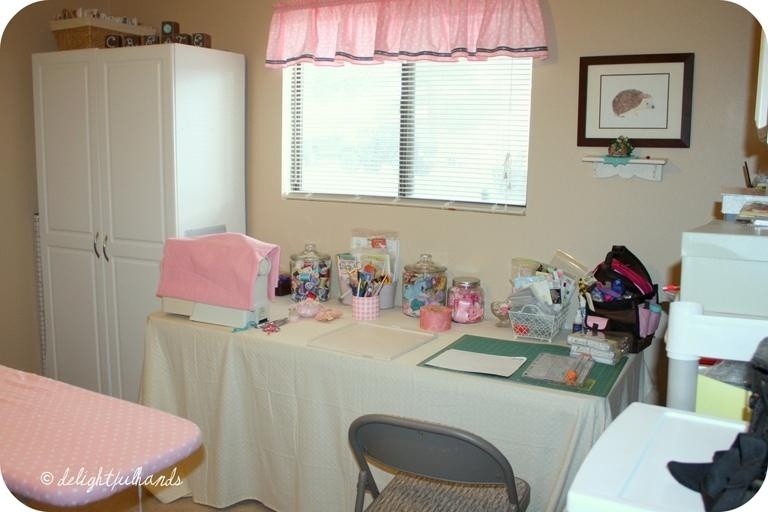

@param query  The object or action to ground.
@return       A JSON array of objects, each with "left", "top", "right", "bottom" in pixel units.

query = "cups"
[{"left": 338, "top": 277, "right": 396, "bottom": 317}]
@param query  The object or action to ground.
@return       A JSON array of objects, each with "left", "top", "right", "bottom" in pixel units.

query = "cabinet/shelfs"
[{"left": 29, "top": 44, "right": 247, "bottom": 403}]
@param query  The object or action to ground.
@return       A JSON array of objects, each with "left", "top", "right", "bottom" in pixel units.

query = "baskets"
[{"left": 507, "top": 303, "right": 572, "bottom": 343}]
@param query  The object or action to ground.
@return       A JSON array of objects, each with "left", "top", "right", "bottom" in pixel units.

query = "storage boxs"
[{"left": 47, "top": 18, "right": 157, "bottom": 50}]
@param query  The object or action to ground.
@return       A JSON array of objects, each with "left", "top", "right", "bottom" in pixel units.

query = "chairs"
[{"left": 346, "top": 413, "right": 532, "bottom": 511}]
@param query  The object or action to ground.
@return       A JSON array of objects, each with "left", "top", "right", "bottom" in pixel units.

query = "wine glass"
[{"left": 491, "top": 301, "right": 511, "bottom": 328}]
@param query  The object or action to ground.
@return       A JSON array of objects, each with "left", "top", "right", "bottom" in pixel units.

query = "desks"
[{"left": 142, "top": 292, "right": 641, "bottom": 510}]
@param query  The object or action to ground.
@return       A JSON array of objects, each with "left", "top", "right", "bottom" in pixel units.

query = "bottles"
[
  {"left": 402, "top": 254, "right": 484, "bottom": 324},
  {"left": 571, "top": 309, "right": 583, "bottom": 334},
  {"left": 289, "top": 243, "right": 333, "bottom": 304}
]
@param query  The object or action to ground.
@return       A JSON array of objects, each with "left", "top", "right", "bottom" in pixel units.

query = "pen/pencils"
[{"left": 351, "top": 271, "right": 387, "bottom": 295}]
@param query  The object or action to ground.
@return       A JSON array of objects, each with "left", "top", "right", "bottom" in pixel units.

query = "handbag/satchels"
[{"left": 582, "top": 246, "right": 661, "bottom": 354}]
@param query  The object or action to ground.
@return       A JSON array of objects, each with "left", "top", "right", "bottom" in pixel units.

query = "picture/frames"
[{"left": 576, "top": 51, "right": 698, "bottom": 150}]
